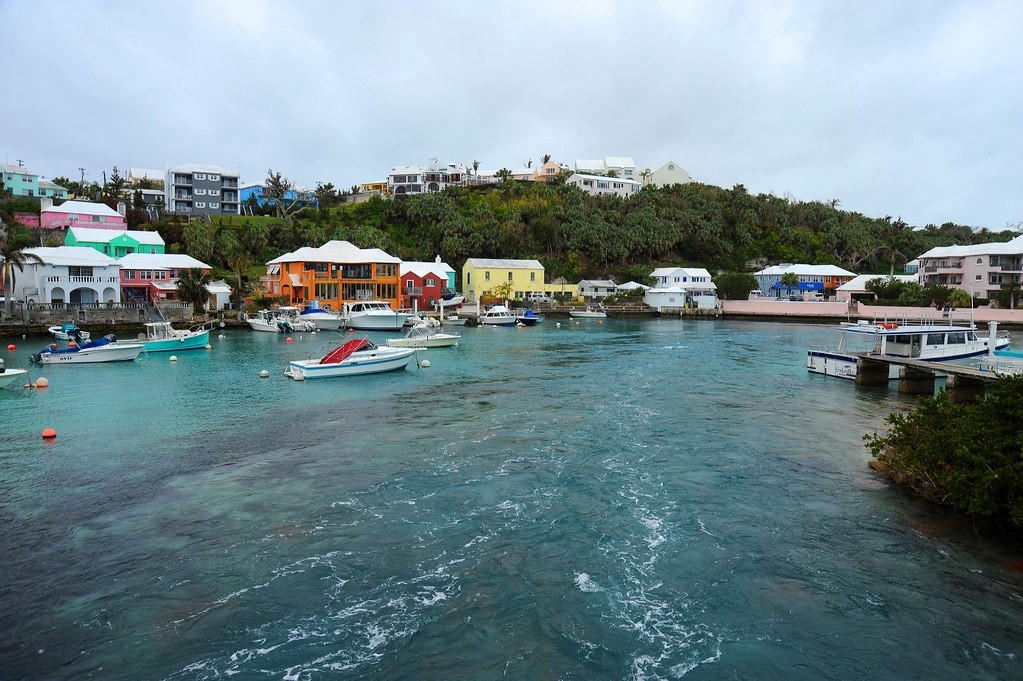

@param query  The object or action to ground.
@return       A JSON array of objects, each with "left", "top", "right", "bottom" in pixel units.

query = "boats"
[
  {"left": 48, "top": 325, "right": 91, "bottom": 340},
  {"left": 568, "top": 302, "right": 608, "bottom": 318},
  {"left": 244, "top": 296, "right": 416, "bottom": 334},
  {"left": 514, "top": 306, "right": 539, "bottom": 326},
  {"left": 430, "top": 288, "right": 465, "bottom": 307},
  {"left": 283, "top": 329, "right": 417, "bottom": 381},
  {"left": 474, "top": 303, "right": 518, "bottom": 327},
  {"left": 0, "top": 358, "right": 28, "bottom": 391},
  {"left": 385, "top": 319, "right": 462, "bottom": 348},
  {"left": 807, "top": 264, "right": 1011, "bottom": 381},
  {"left": 107, "top": 295, "right": 210, "bottom": 352},
  {"left": 28, "top": 319, "right": 146, "bottom": 365}
]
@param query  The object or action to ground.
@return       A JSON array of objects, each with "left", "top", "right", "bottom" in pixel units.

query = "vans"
[{"left": 526, "top": 292, "right": 551, "bottom": 304}]
